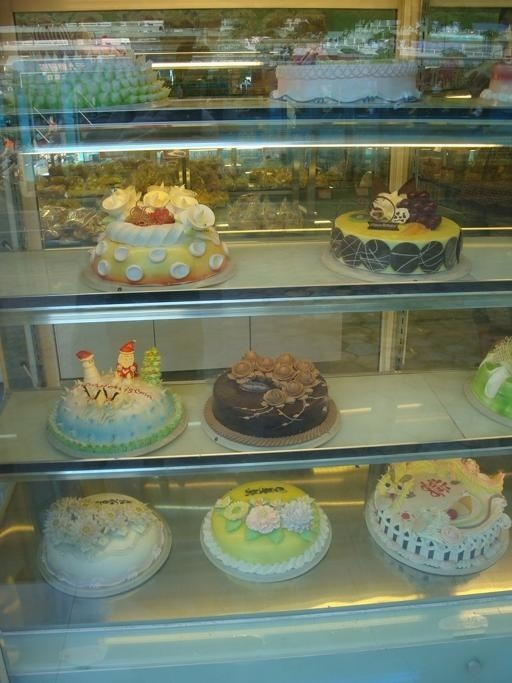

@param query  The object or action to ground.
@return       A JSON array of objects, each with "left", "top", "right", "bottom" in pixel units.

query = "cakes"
[
  {"left": 34, "top": 493, "right": 172, "bottom": 598},
  {"left": 1, "top": 47, "right": 172, "bottom": 116},
  {"left": 272, "top": 60, "right": 422, "bottom": 106},
  {"left": 463, "top": 335, "right": 511, "bottom": 428},
  {"left": 45, "top": 338, "right": 189, "bottom": 458},
  {"left": 326, "top": 189, "right": 463, "bottom": 279},
  {"left": 364, "top": 458, "right": 511, "bottom": 577},
  {"left": 203, "top": 351, "right": 338, "bottom": 448},
  {"left": 200, "top": 480, "right": 332, "bottom": 584},
  {"left": 478, "top": 64, "right": 512, "bottom": 103},
  {"left": 78, "top": 180, "right": 238, "bottom": 293}
]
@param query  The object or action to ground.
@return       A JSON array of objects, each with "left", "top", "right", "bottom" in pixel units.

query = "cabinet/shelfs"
[{"left": 0, "top": 108, "right": 512, "bottom": 681}]
